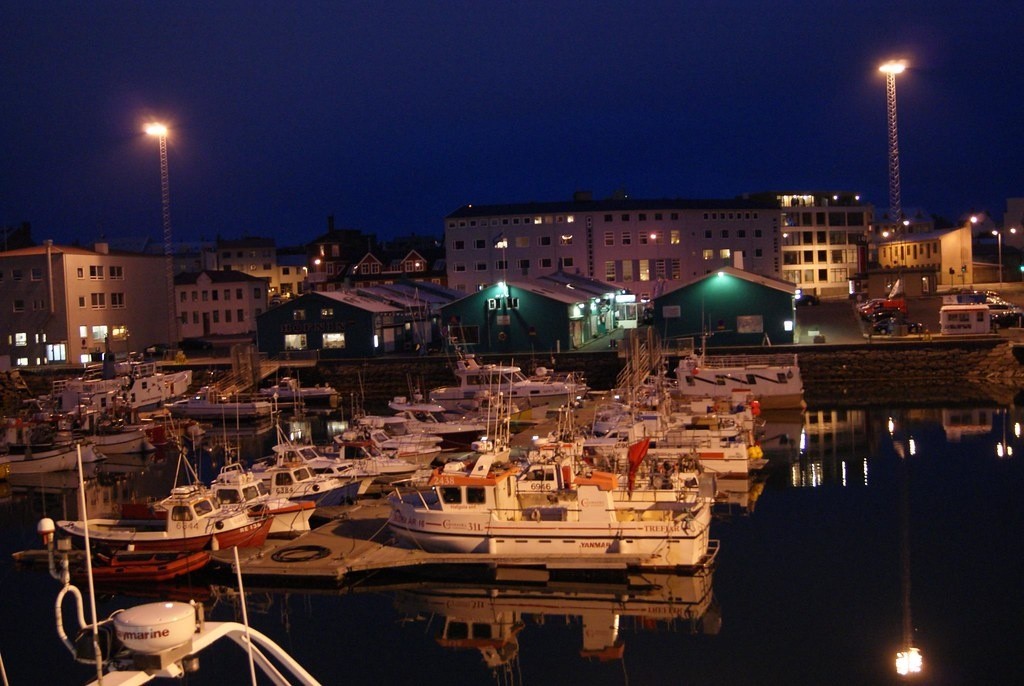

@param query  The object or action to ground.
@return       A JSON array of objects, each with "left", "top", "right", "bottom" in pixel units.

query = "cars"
[
  {"left": 794, "top": 295, "right": 819, "bottom": 307},
  {"left": 857, "top": 296, "right": 929, "bottom": 334},
  {"left": 950, "top": 289, "right": 1024, "bottom": 328}
]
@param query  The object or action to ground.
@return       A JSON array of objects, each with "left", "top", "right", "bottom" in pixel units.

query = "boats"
[{"left": 0, "top": 351, "right": 802, "bottom": 686}]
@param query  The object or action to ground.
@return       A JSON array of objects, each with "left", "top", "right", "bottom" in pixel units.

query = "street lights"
[
  {"left": 496, "top": 241, "right": 507, "bottom": 281},
  {"left": 122, "top": 330, "right": 131, "bottom": 364},
  {"left": 993, "top": 230, "right": 1002, "bottom": 282},
  {"left": 302, "top": 267, "right": 308, "bottom": 289}
]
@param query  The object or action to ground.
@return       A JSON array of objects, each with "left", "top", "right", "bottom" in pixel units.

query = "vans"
[{"left": 860, "top": 296, "right": 906, "bottom": 316}]
